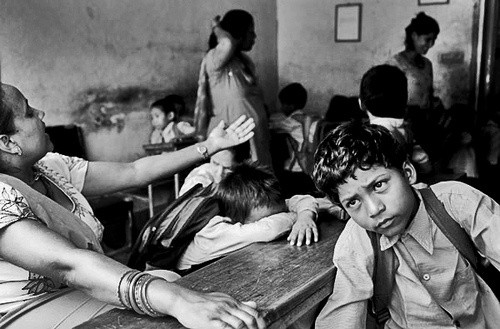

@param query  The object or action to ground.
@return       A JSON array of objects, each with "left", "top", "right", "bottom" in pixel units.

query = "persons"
[
  {"left": 197, "top": 9, "right": 272, "bottom": 174},
  {"left": 384, "top": 11, "right": 500, "bottom": 190},
  {"left": 0, "top": 83, "right": 266, "bottom": 329},
  {"left": 310, "top": 121, "right": 500, "bottom": 329},
  {"left": 268, "top": 82, "right": 311, "bottom": 173},
  {"left": 315, "top": 65, "right": 431, "bottom": 221},
  {"left": 151, "top": 93, "right": 195, "bottom": 144},
  {"left": 177, "top": 150, "right": 239, "bottom": 197},
  {"left": 127, "top": 162, "right": 320, "bottom": 277}
]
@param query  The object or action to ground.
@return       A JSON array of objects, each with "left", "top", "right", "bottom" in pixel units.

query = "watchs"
[{"left": 193, "top": 143, "right": 212, "bottom": 158}]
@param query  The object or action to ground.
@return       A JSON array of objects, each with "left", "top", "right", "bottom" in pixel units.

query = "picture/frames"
[{"left": 335, "top": 2, "right": 362, "bottom": 42}]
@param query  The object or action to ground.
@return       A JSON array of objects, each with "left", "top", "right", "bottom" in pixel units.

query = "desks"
[{"left": 69, "top": 214, "right": 360, "bottom": 329}]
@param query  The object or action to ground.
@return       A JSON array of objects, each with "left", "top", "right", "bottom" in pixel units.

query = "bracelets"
[
  {"left": 212, "top": 22, "right": 220, "bottom": 31},
  {"left": 116, "top": 268, "right": 167, "bottom": 317}
]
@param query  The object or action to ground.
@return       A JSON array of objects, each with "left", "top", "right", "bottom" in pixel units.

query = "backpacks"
[
  {"left": 287, "top": 111, "right": 336, "bottom": 176},
  {"left": 126, "top": 182, "right": 221, "bottom": 272}
]
